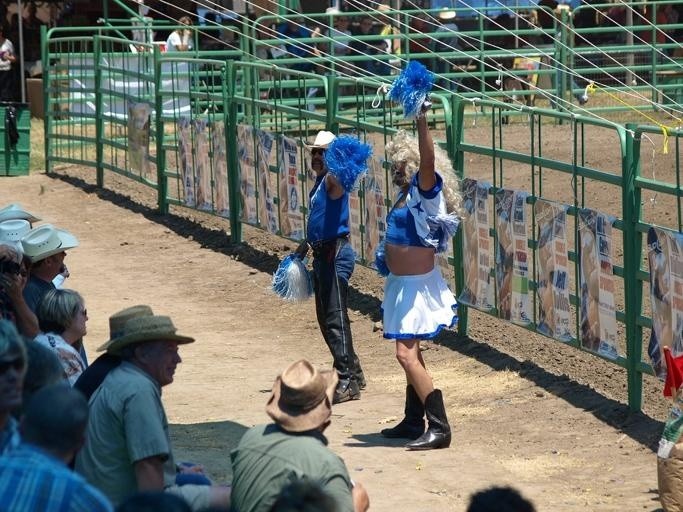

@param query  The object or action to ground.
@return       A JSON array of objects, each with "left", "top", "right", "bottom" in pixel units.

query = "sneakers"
[{"left": 334, "top": 388, "right": 360, "bottom": 404}]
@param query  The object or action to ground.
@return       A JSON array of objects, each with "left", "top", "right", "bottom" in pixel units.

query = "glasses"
[{"left": 311, "top": 148, "right": 324, "bottom": 155}]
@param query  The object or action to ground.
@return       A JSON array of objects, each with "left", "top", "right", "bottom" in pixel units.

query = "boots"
[
  {"left": 381, "top": 384, "right": 425, "bottom": 437},
  {"left": 406, "top": 389, "right": 451, "bottom": 450}
]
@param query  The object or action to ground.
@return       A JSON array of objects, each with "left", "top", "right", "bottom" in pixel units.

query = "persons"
[
  {"left": 229, "top": 359, "right": 369, "bottom": 512},
  {"left": 74, "top": 305, "right": 231, "bottom": 511},
  {"left": 273, "top": 4, "right": 401, "bottom": 111},
  {"left": 165, "top": 16, "right": 193, "bottom": 52},
  {"left": 201, "top": 12, "right": 220, "bottom": 40},
  {"left": 1, "top": 26, "right": 15, "bottom": 99},
  {"left": 273, "top": 129, "right": 374, "bottom": 405},
  {"left": 410, "top": 5, "right": 459, "bottom": 92},
  {"left": 1, "top": 203, "right": 113, "bottom": 510},
  {"left": 371, "top": 63, "right": 466, "bottom": 452},
  {"left": 569, "top": 0, "right": 679, "bottom": 84}
]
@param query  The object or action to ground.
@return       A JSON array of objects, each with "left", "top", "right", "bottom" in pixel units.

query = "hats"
[
  {"left": 301, "top": 130, "right": 337, "bottom": 149},
  {"left": 439, "top": 7, "right": 456, "bottom": 19},
  {"left": 375, "top": 5, "right": 390, "bottom": 11},
  {"left": 325, "top": 7, "right": 340, "bottom": 13},
  {"left": 555, "top": 4, "right": 576, "bottom": 16},
  {"left": 265, "top": 356, "right": 339, "bottom": 433},
  {"left": 0, "top": 203, "right": 196, "bottom": 351}
]
